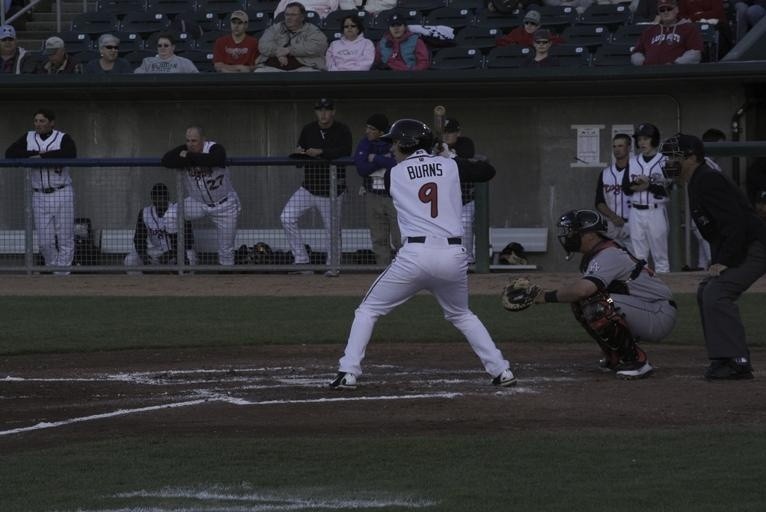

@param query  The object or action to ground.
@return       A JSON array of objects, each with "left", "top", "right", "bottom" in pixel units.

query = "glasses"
[
  {"left": 103, "top": 46, "right": 120, "bottom": 49},
  {"left": 535, "top": 40, "right": 550, "bottom": 44}
]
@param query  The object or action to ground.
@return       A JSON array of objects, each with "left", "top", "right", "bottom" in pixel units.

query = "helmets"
[
  {"left": 380, "top": 119, "right": 433, "bottom": 154},
  {"left": 661, "top": 134, "right": 705, "bottom": 178},
  {"left": 557, "top": 209, "right": 608, "bottom": 261},
  {"left": 632, "top": 123, "right": 661, "bottom": 149}
]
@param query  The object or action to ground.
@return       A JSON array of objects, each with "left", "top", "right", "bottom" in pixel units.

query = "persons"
[
  {"left": 503, "top": 210, "right": 677, "bottom": 380},
  {"left": 658, "top": 133, "right": 766, "bottom": 380},
  {"left": 331, "top": 119, "right": 516, "bottom": 389}
]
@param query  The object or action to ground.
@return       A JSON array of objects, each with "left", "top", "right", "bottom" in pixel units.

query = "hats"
[
  {"left": 389, "top": 13, "right": 405, "bottom": 25},
  {"left": 523, "top": 10, "right": 542, "bottom": 24},
  {"left": 444, "top": 119, "right": 460, "bottom": 133},
  {"left": 42, "top": 36, "right": 64, "bottom": 56},
  {"left": 313, "top": 98, "right": 334, "bottom": 109},
  {"left": 532, "top": 28, "right": 551, "bottom": 40},
  {"left": 0, "top": 25, "right": 17, "bottom": 41},
  {"left": 229, "top": 10, "right": 249, "bottom": 23},
  {"left": 657, "top": 1, "right": 678, "bottom": 8}
]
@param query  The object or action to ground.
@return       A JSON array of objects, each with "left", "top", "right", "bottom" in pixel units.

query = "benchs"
[{"left": 2, "top": 227, "right": 550, "bottom": 256}]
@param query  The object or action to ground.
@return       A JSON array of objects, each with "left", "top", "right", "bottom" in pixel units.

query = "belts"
[
  {"left": 632, "top": 203, "right": 658, "bottom": 209},
  {"left": 407, "top": 236, "right": 461, "bottom": 245},
  {"left": 208, "top": 197, "right": 228, "bottom": 207},
  {"left": 33, "top": 186, "right": 65, "bottom": 193}
]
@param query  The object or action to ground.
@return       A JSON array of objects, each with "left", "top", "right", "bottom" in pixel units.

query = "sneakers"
[
  {"left": 598, "top": 356, "right": 615, "bottom": 372},
  {"left": 615, "top": 361, "right": 651, "bottom": 379},
  {"left": 705, "top": 359, "right": 753, "bottom": 381},
  {"left": 490, "top": 369, "right": 517, "bottom": 387},
  {"left": 330, "top": 372, "right": 359, "bottom": 390}
]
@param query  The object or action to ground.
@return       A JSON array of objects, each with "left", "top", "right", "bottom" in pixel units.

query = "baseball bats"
[{"left": 434, "top": 106, "right": 445, "bottom": 137}]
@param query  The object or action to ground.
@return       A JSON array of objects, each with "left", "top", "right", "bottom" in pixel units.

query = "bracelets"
[{"left": 544, "top": 289, "right": 559, "bottom": 303}]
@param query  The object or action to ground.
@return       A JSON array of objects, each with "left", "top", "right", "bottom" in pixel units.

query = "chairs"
[{"left": 2, "top": 1, "right": 764, "bottom": 73}]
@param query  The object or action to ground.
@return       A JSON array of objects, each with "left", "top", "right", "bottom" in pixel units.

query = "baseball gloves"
[{"left": 500, "top": 277, "right": 544, "bottom": 311}]
